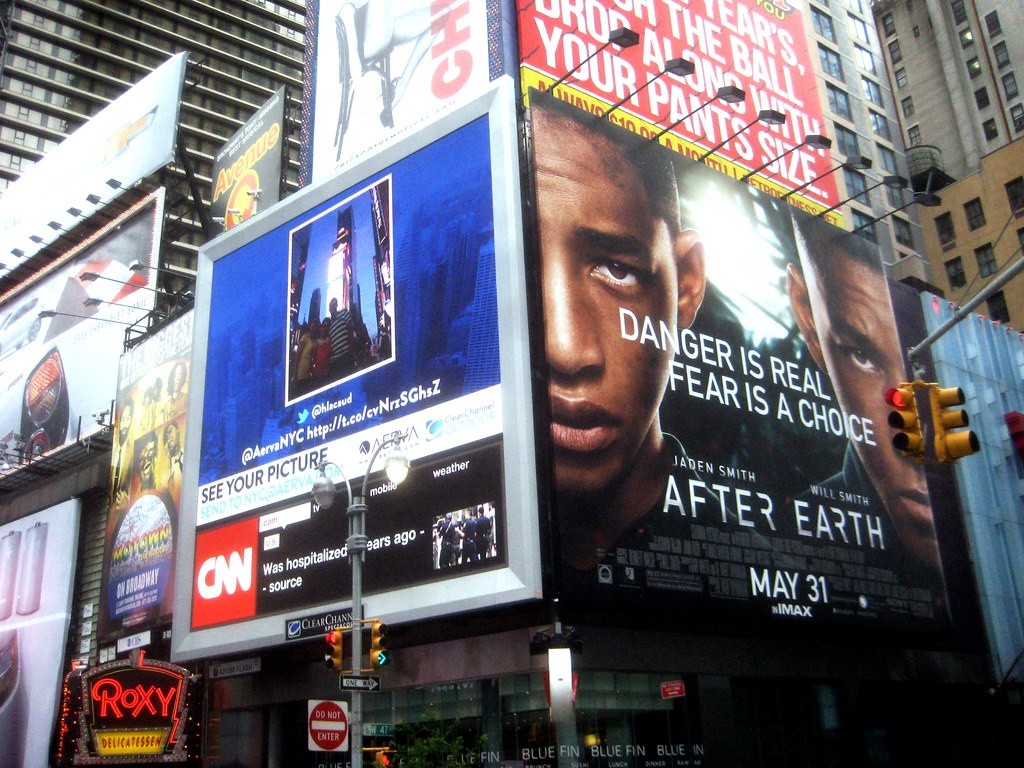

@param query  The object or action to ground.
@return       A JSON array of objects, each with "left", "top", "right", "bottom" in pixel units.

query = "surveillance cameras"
[{"left": 92, "top": 409, "right": 109, "bottom": 417}]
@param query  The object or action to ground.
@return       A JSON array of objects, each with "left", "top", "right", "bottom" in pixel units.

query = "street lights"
[{"left": 311, "top": 433, "right": 412, "bottom": 768}]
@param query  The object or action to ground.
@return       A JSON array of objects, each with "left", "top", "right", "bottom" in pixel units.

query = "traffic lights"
[
  {"left": 370, "top": 623, "right": 391, "bottom": 669},
  {"left": 884, "top": 382, "right": 923, "bottom": 457},
  {"left": 928, "top": 385, "right": 980, "bottom": 465},
  {"left": 325, "top": 630, "right": 343, "bottom": 672}
]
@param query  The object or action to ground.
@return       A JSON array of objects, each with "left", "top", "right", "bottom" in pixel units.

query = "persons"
[
  {"left": 292, "top": 315, "right": 318, "bottom": 399},
  {"left": 313, "top": 318, "right": 331, "bottom": 388},
  {"left": 326, "top": 298, "right": 353, "bottom": 384},
  {"left": 110, "top": 361, "right": 189, "bottom": 510},
  {"left": 786, "top": 209, "right": 942, "bottom": 567},
  {"left": 529, "top": 89, "right": 820, "bottom": 628},
  {"left": 438, "top": 507, "right": 491, "bottom": 568}
]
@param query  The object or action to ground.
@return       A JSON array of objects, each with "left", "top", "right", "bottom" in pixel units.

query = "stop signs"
[{"left": 307, "top": 699, "right": 349, "bottom": 752}]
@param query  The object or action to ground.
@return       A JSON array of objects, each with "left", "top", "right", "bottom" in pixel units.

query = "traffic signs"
[{"left": 339, "top": 674, "right": 382, "bottom": 694}]
